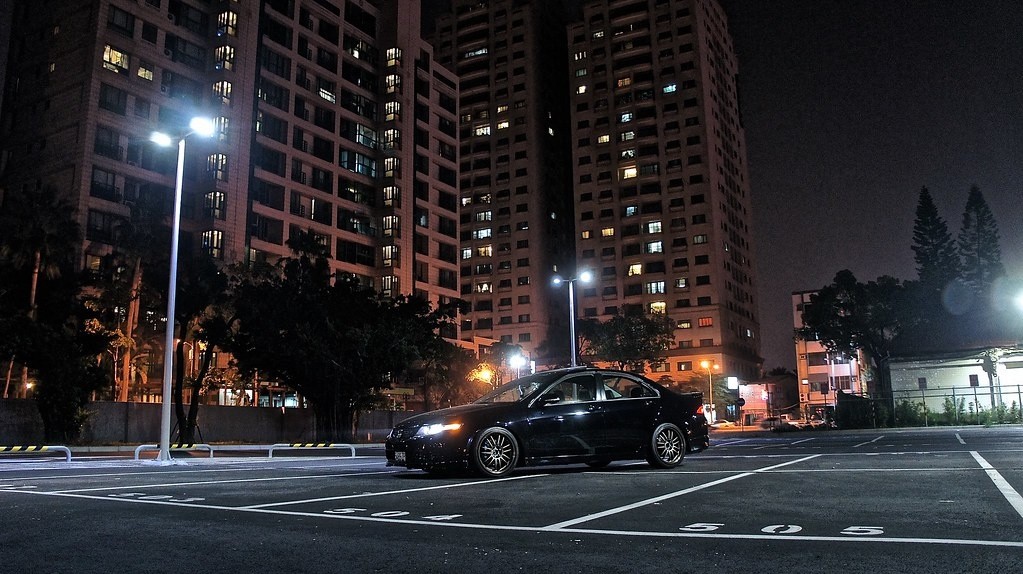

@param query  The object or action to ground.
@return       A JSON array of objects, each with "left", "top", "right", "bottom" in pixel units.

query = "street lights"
[
  {"left": 554, "top": 271, "right": 592, "bottom": 401},
  {"left": 512, "top": 356, "right": 524, "bottom": 390},
  {"left": 824, "top": 357, "right": 844, "bottom": 412},
  {"left": 702, "top": 361, "right": 719, "bottom": 422},
  {"left": 149, "top": 115, "right": 211, "bottom": 461}
]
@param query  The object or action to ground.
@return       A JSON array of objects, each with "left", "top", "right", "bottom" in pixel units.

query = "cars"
[
  {"left": 708, "top": 419, "right": 735, "bottom": 430},
  {"left": 761, "top": 416, "right": 789, "bottom": 430},
  {"left": 385, "top": 363, "right": 710, "bottom": 479},
  {"left": 784, "top": 416, "right": 838, "bottom": 429}
]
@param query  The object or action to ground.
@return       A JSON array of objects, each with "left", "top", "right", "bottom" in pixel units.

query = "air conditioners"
[{"left": 800, "top": 393, "right": 808, "bottom": 402}]
